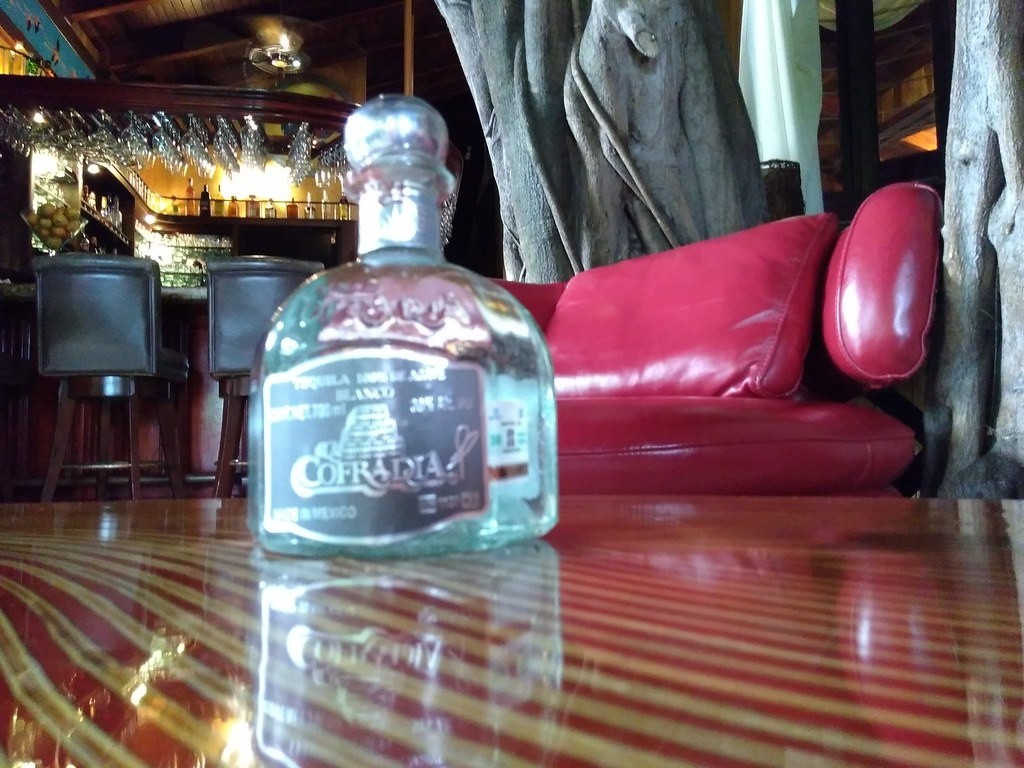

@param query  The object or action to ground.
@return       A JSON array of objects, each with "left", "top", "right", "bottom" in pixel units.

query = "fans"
[{"left": 249, "top": 35, "right": 308, "bottom": 75}]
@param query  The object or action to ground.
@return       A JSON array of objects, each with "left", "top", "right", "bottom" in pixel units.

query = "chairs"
[
  {"left": 207, "top": 256, "right": 325, "bottom": 500},
  {"left": 33, "top": 252, "right": 190, "bottom": 500}
]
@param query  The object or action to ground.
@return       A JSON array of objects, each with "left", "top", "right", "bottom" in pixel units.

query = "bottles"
[
  {"left": 228, "top": 196, "right": 240, "bottom": 217},
  {"left": 246, "top": 195, "right": 260, "bottom": 219},
  {"left": 287, "top": 198, "right": 298, "bottom": 219},
  {"left": 170, "top": 195, "right": 181, "bottom": 215},
  {"left": 214, "top": 185, "right": 225, "bottom": 216},
  {"left": 198, "top": 183, "right": 211, "bottom": 216},
  {"left": 334, "top": 192, "right": 350, "bottom": 220},
  {"left": 79, "top": 184, "right": 124, "bottom": 255},
  {"left": 183, "top": 176, "right": 196, "bottom": 215},
  {"left": 303, "top": 192, "right": 317, "bottom": 220},
  {"left": 234, "top": 94, "right": 561, "bottom": 558},
  {"left": 265, "top": 198, "right": 276, "bottom": 218},
  {"left": 320, "top": 189, "right": 332, "bottom": 220}
]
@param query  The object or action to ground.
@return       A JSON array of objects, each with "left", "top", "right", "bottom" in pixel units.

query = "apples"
[{"left": 26, "top": 203, "right": 81, "bottom": 249}]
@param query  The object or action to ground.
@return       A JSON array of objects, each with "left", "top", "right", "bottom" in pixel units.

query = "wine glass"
[{"left": 1, "top": 102, "right": 348, "bottom": 188}]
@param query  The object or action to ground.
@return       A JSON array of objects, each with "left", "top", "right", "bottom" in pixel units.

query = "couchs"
[{"left": 489, "top": 181, "right": 943, "bottom": 496}]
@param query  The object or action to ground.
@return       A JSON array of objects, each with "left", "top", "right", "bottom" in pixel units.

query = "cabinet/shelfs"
[{"left": 0, "top": 146, "right": 137, "bottom": 284}]
